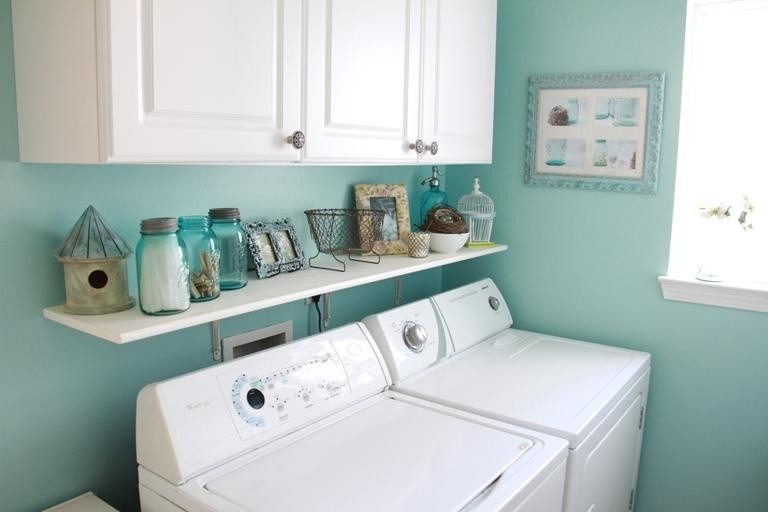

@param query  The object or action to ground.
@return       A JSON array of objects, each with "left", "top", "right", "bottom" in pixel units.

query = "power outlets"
[{"left": 304, "top": 295, "right": 323, "bottom": 304}]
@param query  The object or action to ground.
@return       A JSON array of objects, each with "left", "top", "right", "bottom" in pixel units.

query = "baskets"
[{"left": 305, "top": 208, "right": 386, "bottom": 254}]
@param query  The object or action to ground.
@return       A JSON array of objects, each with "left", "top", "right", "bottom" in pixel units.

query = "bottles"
[
  {"left": 134, "top": 217, "right": 190, "bottom": 316},
  {"left": 177, "top": 214, "right": 221, "bottom": 303},
  {"left": 208, "top": 207, "right": 248, "bottom": 292},
  {"left": 593, "top": 139, "right": 607, "bottom": 168},
  {"left": 566, "top": 98, "right": 580, "bottom": 125}
]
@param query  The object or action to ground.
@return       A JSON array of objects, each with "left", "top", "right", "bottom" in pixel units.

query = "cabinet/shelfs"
[{"left": 11, "top": 0, "right": 499, "bottom": 169}]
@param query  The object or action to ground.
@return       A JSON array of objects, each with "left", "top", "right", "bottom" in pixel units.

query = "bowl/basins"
[{"left": 425, "top": 229, "right": 470, "bottom": 254}]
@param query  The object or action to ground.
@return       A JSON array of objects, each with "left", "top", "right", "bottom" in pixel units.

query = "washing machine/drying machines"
[
  {"left": 135, "top": 320, "right": 569, "bottom": 512},
  {"left": 361, "top": 276, "right": 653, "bottom": 512}
]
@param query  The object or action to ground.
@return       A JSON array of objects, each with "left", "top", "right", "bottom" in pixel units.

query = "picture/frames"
[
  {"left": 353, "top": 182, "right": 412, "bottom": 257},
  {"left": 242, "top": 217, "right": 308, "bottom": 280},
  {"left": 521, "top": 69, "right": 667, "bottom": 195}
]
